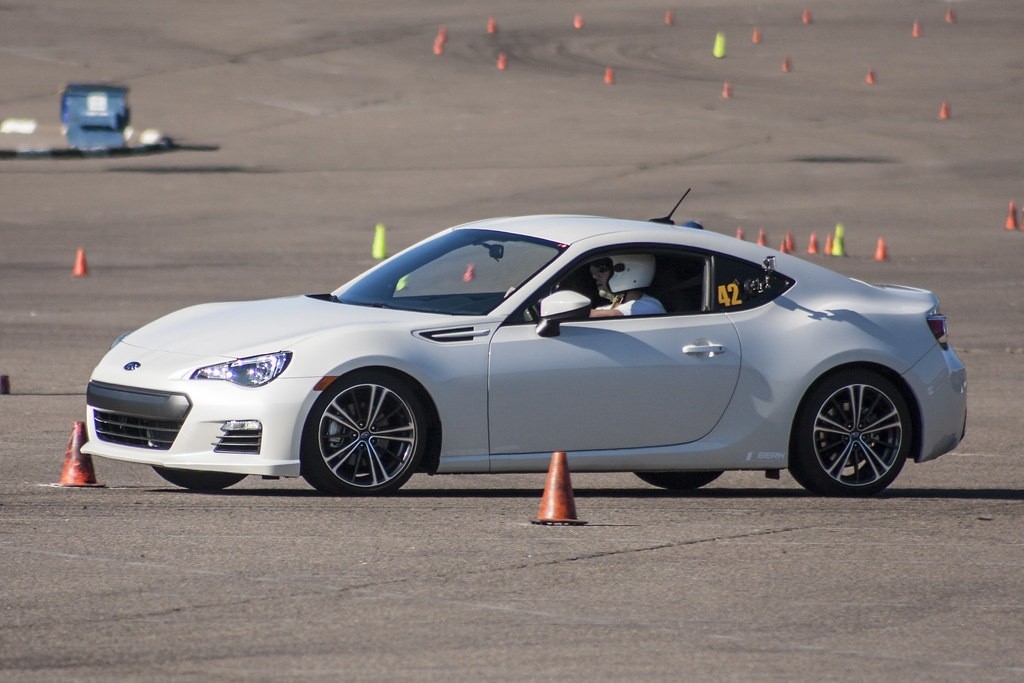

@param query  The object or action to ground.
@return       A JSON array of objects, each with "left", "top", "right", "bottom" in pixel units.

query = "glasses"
[{"left": 598, "top": 263, "right": 611, "bottom": 272}]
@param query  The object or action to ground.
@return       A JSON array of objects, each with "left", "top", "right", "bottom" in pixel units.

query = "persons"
[{"left": 588, "top": 252, "right": 666, "bottom": 317}]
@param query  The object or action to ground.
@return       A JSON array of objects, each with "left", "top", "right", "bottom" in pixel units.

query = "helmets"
[{"left": 591, "top": 256, "right": 655, "bottom": 294}]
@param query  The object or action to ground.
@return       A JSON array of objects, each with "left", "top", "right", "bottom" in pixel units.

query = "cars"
[{"left": 78, "top": 212, "right": 969, "bottom": 498}]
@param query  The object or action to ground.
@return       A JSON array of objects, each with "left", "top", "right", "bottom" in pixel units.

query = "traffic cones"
[
  {"left": 722, "top": 82, "right": 733, "bottom": 99},
  {"left": 56, "top": 420, "right": 106, "bottom": 488},
  {"left": 945, "top": 7, "right": 955, "bottom": 23},
  {"left": 371, "top": 223, "right": 386, "bottom": 259},
  {"left": 573, "top": 13, "right": 583, "bottom": 29},
  {"left": 939, "top": 98, "right": 950, "bottom": 119},
  {"left": 487, "top": 18, "right": 497, "bottom": 34},
  {"left": 432, "top": 25, "right": 449, "bottom": 54},
  {"left": 604, "top": 67, "right": 615, "bottom": 83},
  {"left": 72, "top": 247, "right": 88, "bottom": 276},
  {"left": 736, "top": 222, "right": 846, "bottom": 257},
  {"left": 712, "top": 8, "right": 812, "bottom": 72},
  {"left": 874, "top": 235, "right": 887, "bottom": 261},
  {"left": 664, "top": 9, "right": 674, "bottom": 25},
  {"left": 497, "top": 53, "right": 507, "bottom": 70},
  {"left": 912, "top": 17, "right": 922, "bottom": 37},
  {"left": 1004, "top": 200, "right": 1024, "bottom": 231},
  {"left": 865, "top": 71, "right": 876, "bottom": 85},
  {"left": 529, "top": 449, "right": 589, "bottom": 527}
]
